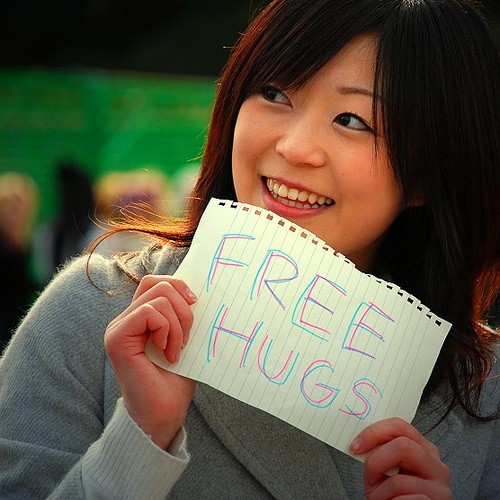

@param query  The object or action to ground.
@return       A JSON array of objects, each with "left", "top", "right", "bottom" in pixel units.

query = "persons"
[
  {"left": 1, "top": 0, "right": 500, "bottom": 500},
  {"left": 1, "top": 160, "right": 170, "bottom": 358}
]
